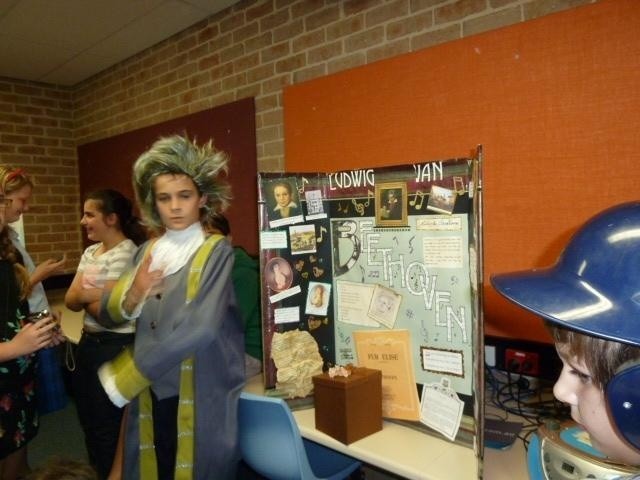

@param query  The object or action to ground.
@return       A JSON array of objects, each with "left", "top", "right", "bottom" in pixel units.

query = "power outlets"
[{"left": 485, "top": 345, "right": 540, "bottom": 392}]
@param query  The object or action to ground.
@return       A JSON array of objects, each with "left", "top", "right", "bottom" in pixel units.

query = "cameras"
[{"left": 26, "top": 309, "right": 61, "bottom": 332}]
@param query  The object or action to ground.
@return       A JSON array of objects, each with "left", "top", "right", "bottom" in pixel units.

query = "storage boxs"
[{"left": 312, "top": 364, "right": 385, "bottom": 447}]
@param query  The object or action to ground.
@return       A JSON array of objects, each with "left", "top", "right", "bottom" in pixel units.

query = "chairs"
[{"left": 234, "top": 391, "right": 364, "bottom": 480}]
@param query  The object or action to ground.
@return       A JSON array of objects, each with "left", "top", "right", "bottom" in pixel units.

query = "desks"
[{"left": 240, "top": 362, "right": 486, "bottom": 478}]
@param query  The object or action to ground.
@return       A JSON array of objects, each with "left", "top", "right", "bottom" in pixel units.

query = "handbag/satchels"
[{"left": 60, "top": 307, "right": 85, "bottom": 345}]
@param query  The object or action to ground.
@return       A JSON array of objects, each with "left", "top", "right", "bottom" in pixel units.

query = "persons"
[
  {"left": 267, "top": 179, "right": 300, "bottom": 220},
  {"left": 0, "top": 163, "right": 98, "bottom": 479},
  {"left": 63, "top": 187, "right": 140, "bottom": 479},
  {"left": 310, "top": 285, "right": 324, "bottom": 307},
  {"left": 268, "top": 260, "right": 291, "bottom": 291},
  {"left": 382, "top": 189, "right": 399, "bottom": 219},
  {"left": 201, "top": 210, "right": 262, "bottom": 362},
  {"left": 97, "top": 135, "right": 246, "bottom": 480}
]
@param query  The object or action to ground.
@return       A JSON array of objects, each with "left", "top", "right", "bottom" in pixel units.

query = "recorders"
[{"left": 527, "top": 419, "right": 640, "bottom": 480}]
